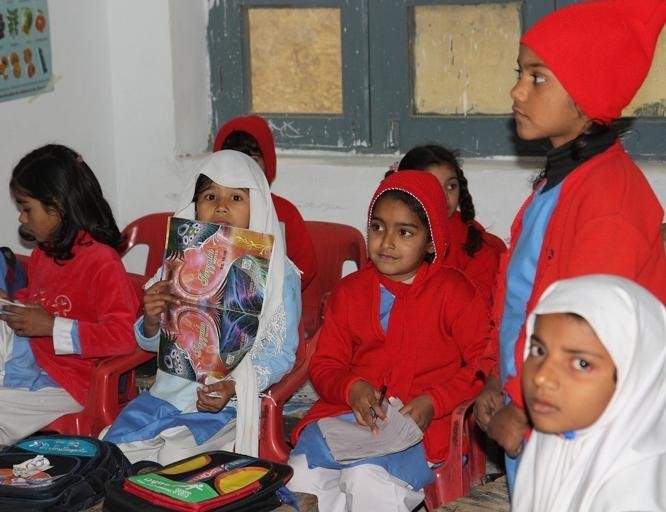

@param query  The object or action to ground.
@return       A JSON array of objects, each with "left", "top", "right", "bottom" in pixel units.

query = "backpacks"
[
  {"left": 101, "top": 449, "right": 301, "bottom": 512},
  {"left": 0, "top": 434, "right": 131, "bottom": 512}
]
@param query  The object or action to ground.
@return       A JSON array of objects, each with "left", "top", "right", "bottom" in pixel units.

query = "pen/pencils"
[{"left": 373, "top": 385, "right": 388, "bottom": 426}]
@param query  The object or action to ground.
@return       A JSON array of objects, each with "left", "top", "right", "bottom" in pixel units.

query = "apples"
[{"left": 36, "top": 8, "right": 45, "bottom": 32}]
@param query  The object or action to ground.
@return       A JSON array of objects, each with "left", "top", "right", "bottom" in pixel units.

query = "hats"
[{"left": 520, "top": 0, "right": 666, "bottom": 124}]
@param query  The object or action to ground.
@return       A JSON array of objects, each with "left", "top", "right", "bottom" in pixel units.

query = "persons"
[
  {"left": 210, "top": 114, "right": 317, "bottom": 291},
  {"left": 509, "top": 272, "right": 666, "bottom": 512},
  {"left": 379, "top": 144, "right": 507, "bottom": 313},
  {"left": 0, "top": 144, "right": 141, "bottom": 447},
  {"left": 95, "top": 148, "right": 304, "bottom": 467},
  {"left": 286, "top": 171, "right": 494, "bottom": 512},
  {"left": 472, "top": 0, "right": 666, "bottom": 507}
]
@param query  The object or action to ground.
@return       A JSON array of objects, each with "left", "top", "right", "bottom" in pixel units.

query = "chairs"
[
  {"left": 424, "top": 227, "right": 506, "bottom": 510},
  {"left": 260, "top": 220, "right": 368, "bottom": 464},
  {"left": 38, "top": 213, "right": 168, "bottom": 436}
]
